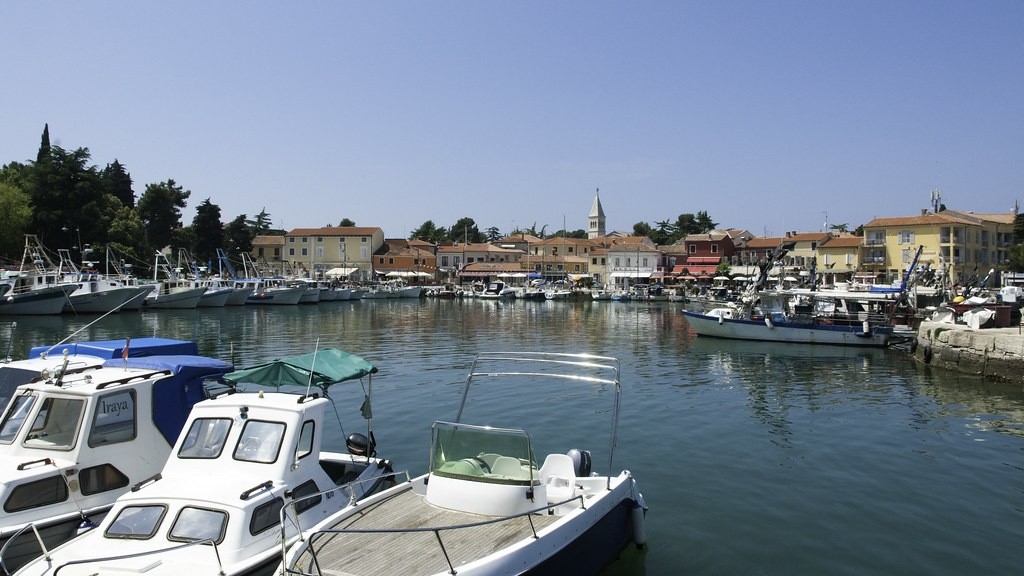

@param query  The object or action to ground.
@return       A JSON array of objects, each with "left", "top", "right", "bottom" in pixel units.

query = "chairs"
[
  {"left": 491, "top": 456, "right": 522, "bottom": 478},
  {"left": 536, "top": 453, "right": 577, "bottom": 504}
]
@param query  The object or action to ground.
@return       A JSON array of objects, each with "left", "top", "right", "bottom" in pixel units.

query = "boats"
[
  {"left": 0, "top": 226, "right": 1023, "bottom": 348},
  {"left": 267, "top": 351, "right": 648, "bottom": 576},
  {"left": 0, "top": 336, "right": 200, "bottom": 436},
  {"left": 0, "top": 356, "right": 236, "bottom": 561},
  {"left": 0, "top": 348, "right": 396, "bottom": 576}
]
